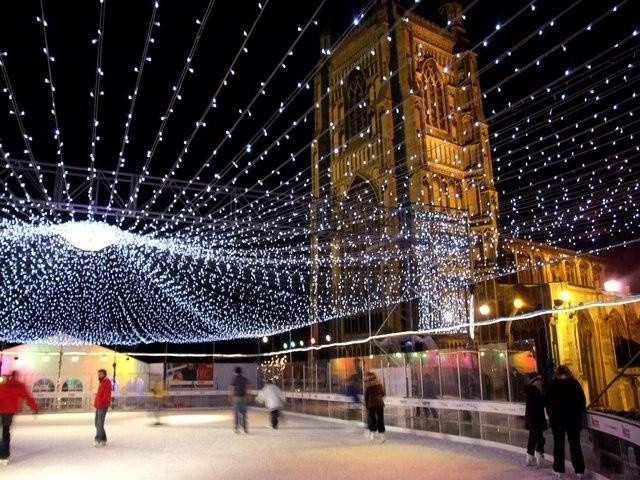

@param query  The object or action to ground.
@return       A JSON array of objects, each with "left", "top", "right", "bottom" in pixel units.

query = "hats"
[{"left": 527, "top": 371, "right": 541, "bottom": 385}]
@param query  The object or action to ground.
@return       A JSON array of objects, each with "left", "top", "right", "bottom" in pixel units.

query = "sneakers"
[{"left": 525, "top": 451, "right": 587, "bottom": 479}]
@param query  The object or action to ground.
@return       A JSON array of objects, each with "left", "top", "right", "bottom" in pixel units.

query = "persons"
[
  {"left": 227, "top": 366, "right": 250, "bottom": 433},
  {"left": 166, "top": 363, "right": 199, "bottom": 389},
  {"left": 0, "top": 369, "right": 40, "bottom": 460},
  {"left": 523, "top": 370, "right": 553, "bottom": 468},
  {"left": 148, "top": 374, "right": 165, "bottom": 425},
  {"left": 349, "top": 356, "right": 365, "bottom": 380},
  {"left": 93, "top": 369, "right": 112, "bottom": 444},
  {"left": 255, "top": 377, "right": 287, "bottom": 430},
  {"left": 364, "top": 372, "right": 387, "bottom": 442},
  {"left": 343, "top": 376, "right": 363, "bottom": 421},
  {"left": 544, "top": 365, "right": 592, "bottom": 479},
  {"left": 405, "top": 366, "right": 529, "bottom": 433}
]
